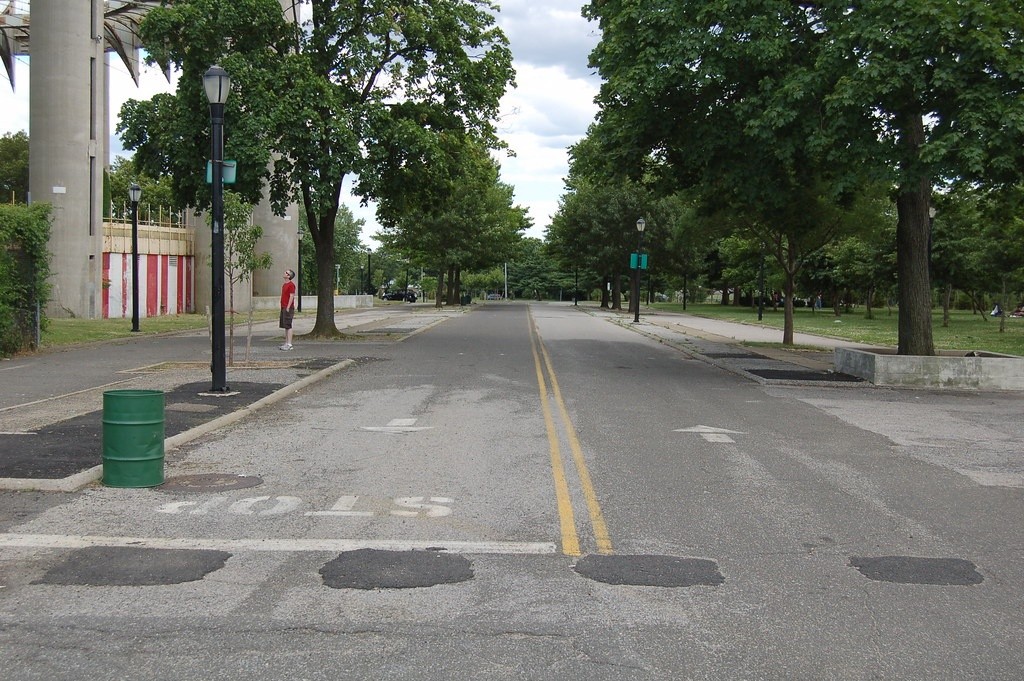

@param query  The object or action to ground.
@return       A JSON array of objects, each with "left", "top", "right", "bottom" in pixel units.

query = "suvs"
[{"left": 382, "top": 289, "right": 417, "bottom": 303}]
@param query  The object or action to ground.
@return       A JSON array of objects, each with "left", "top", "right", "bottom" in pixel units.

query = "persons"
[
  {"left": 793, "top": 295, "right": 824, "bottom": 309},
  {"left": 279, "top": 269, "right": 295, "bottom": 350},
  {"left": 1010, "top": 306, "right": 1024, "bottom": 316}
]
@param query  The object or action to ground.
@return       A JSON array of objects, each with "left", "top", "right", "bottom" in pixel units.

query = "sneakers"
[{"left": 280, "top": 344, "right": 294, "bottom": 351}]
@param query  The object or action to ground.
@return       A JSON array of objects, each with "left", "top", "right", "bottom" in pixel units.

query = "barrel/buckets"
[{"left": 100, "top": 389, "right": 165, "bottom": 487}]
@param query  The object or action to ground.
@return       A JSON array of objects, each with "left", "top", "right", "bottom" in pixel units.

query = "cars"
[
  {"left": 657, "top": 295, "right": 672, "bottom": 302},
  {"left": 488, "top": 294, "right": 503, "bottom": 300}
]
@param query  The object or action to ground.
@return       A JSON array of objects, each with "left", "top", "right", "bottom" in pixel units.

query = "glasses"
[{"left": 286, "top": 271, "right": 291, "bottom": 276}]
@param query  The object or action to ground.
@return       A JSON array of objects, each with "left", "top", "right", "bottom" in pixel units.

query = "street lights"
[
  {"left": 634, "top": 219, "right": 646, "bottom": 322},
  {"left": 201, "top": 65, "right": 231, "bottom": 394},
  {"left": 360, "top": 265, "right": 364, "bottom": 295},
  {"left": 335, "top": 264, "right": 341, "bottom": 295},
  {"left": 129, "top": 185, "right": 141, "bottom": 331},
  {"left": 367, "top": 248, "right": 372, "bottom": 294}
]
